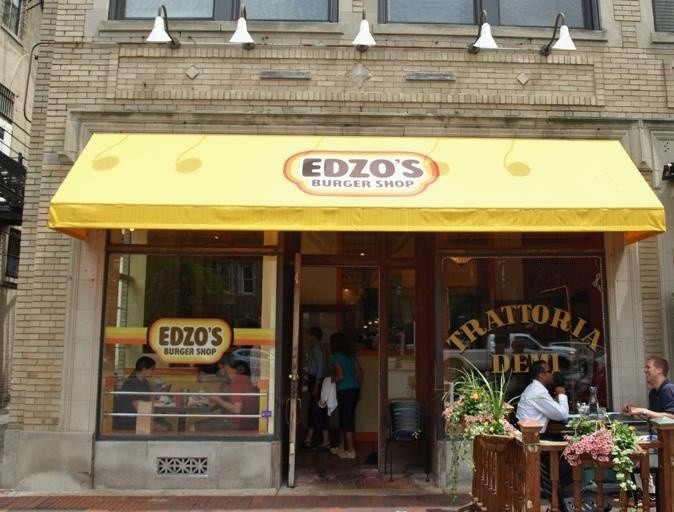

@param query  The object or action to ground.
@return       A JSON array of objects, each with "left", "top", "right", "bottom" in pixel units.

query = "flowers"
[
  {"left": 436, "top": 348, "right": 525, "bottom": 507},
  {"left": 557, "top": 402, "right": 652, "bottom": 512}
]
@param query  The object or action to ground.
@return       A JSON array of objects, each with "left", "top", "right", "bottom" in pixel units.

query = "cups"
[
  {"left": 598, "top": 407, "right": 606, "bottom": 420},
  {"left": 576, "top": 402, "right": 589, "bottom": 417}
]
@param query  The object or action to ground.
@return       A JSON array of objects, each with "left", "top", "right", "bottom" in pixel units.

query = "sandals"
[{"left": 301, "top": 440, "right": 331, "bottom": 452}]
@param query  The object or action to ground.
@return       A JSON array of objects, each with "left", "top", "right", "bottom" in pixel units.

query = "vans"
[{"left": 548, "top": 339, "right": 605, "bottom": 378}]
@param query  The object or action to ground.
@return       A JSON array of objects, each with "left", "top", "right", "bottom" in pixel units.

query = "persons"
[
  {"left": 622, "top": 357, "right": 673, "bottom": 507},
  {"left": 328, "top": 331, "right": 364, "bottom": 459},
  {"left": 514, "top": 359, "right": 573, "bottom": 497},
  {"left": 300, "top": 325, "right": 331, "bottom": 452},
  {"left": 187, "top": 352, "right": 260, "bottom": 431},
  {"left": 112, "top": 356, "right": 174, "bottom": 433},
  {"left": 211, "top": 354, "right": 236, "bottom": 415}
]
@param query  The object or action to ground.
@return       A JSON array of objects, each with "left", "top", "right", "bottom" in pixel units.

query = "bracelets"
[
  {"left": 315, "top": 379, "right": 322, "bottom": 384},
  {"left": 643, "top": 408, "right": 648, "bottom": 416},
  {"left": 558, "top": 391, "right": 566, "bottom": 394}
]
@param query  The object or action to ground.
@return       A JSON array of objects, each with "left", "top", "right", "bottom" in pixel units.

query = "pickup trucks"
[{"left": 443, "top": 330, "right": 577, "bottom": 379}]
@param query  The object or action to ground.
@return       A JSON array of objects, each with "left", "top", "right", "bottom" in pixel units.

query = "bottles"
[
  {"left": 176, "top": 386, "right": 189, "bottom": 406},
  {"left": 197, "top": 382, "right": 205, "bottom": 398},
  {"left": 588, "top": 386, "right": 599, "bottom": 420}
]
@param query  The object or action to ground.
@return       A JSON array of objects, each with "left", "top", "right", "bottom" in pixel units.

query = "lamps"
[
  {"left": 537, "top": 10, "right": 579, "bottom": 58},
  {"left": 144, "top": 4, "right": 181, "bottom": 51},
  {"left": 464, "top": 8, "right": 500, "bottom": 59},
  {"left": 350, "top": 9, "right": 377, "bottom": 53},
  {"left": 226, "top": 5, "right": 257, "bottom": 52}
]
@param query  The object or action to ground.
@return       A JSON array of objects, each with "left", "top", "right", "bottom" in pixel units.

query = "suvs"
[{"left": 229, "top": 348, "right": 273, "bottom": 376}]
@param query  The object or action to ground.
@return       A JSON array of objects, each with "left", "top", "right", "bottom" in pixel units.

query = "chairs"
[{"left": 381, "top": 395, "right": 436, "bottom": 486}]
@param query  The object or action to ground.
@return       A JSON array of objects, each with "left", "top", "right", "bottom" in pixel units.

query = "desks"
[
  {"left": 560, "top": 410, "right": 648, "bottom": 432},
  {"left": 149, "top": 402, "right": 222, "bottom": 434}
]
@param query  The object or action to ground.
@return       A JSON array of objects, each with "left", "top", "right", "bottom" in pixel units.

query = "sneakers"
[{"left": 330, "top": 447, "right": 357, "bottom": 459}]
